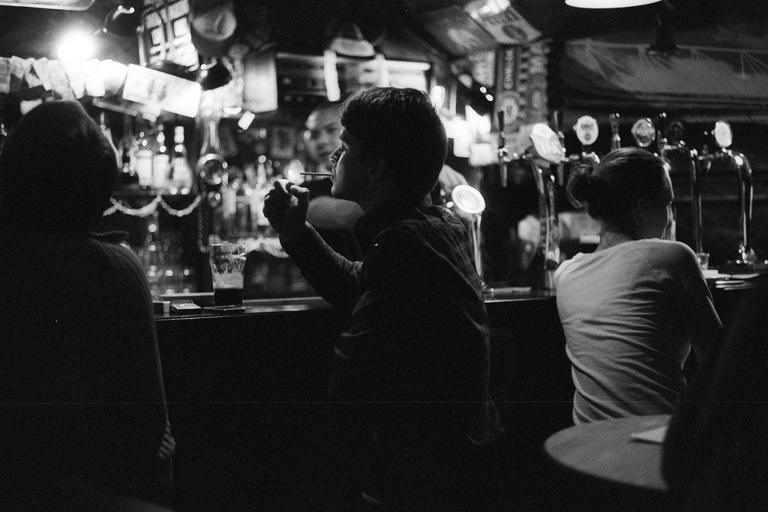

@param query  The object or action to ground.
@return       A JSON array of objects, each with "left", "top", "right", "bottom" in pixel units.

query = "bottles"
[
  {"left": 224, "top": 150, "right": 291, "bottom": 239},
  {"left": 99, "top": 105, "right": 197, "bottom": 301}
]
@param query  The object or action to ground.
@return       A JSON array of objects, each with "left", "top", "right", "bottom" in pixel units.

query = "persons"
[
  {"left": 550, "top": 145, "right": 726, "bottom": 426},
  {"left": 0, "top": 100, "right": 177, "bottom": 512},
  {"left": 297, "top": 98, "right": 364, "bottom": 263},
  {"left": 260, "top": 86, "right": 489, "bottom": 512}
]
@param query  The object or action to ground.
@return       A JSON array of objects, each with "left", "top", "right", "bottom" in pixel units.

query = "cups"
[
  {"left": 209, "top": 241, "right": 247, "bottom": 308},
  {"left": 695, "top": 251, "right": 712, "bottom": 273}
]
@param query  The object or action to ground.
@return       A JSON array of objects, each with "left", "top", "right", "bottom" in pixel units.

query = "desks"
[{"left": 541, "top": 413, "right": 680, "bottom": 500}]
